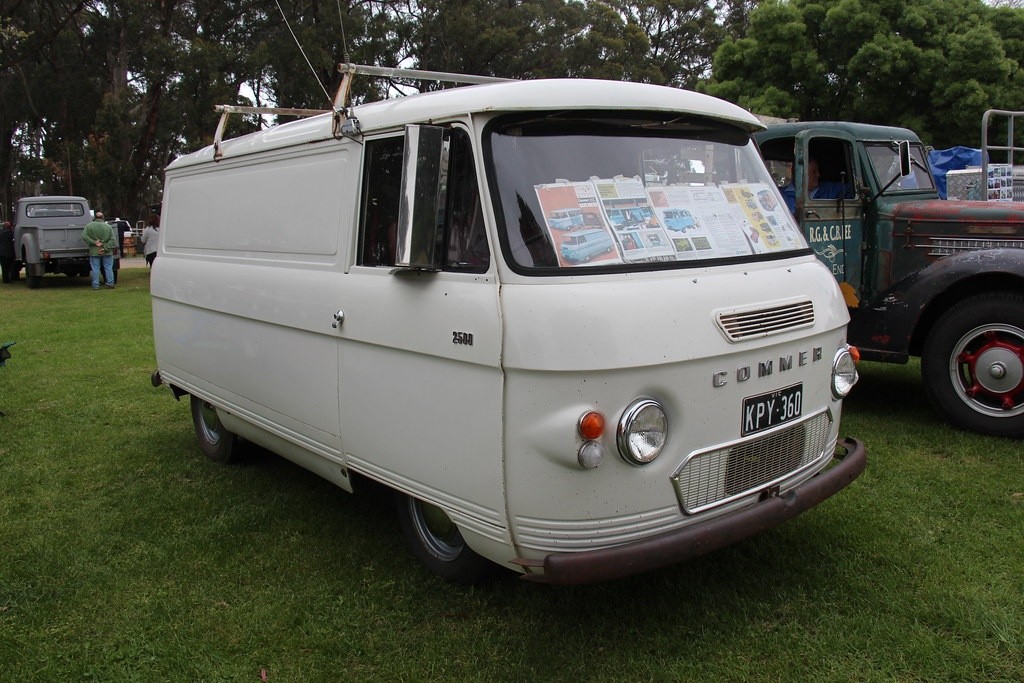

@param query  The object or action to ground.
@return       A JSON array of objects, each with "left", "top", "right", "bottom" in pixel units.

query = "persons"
[
  {"left": 81, "top": 212, "right": 116, "bottom": 290},
  {"left": 0, "top": 221, "right": 20, "bottom": 284},
  {"left": 140, "top": 214, "right": 161, "bottom": 268},
  {"left": 779, "top": 157, "right": 850, "bottom": 219}
]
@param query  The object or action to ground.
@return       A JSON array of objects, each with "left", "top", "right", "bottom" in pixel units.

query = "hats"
[{"left": 4, "top": 221, "right": 11, "bottom": 227}]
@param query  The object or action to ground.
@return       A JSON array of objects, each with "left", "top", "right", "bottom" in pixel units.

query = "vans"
[{"left": 149, "top": 64, "right": 870, "bottom": 588}]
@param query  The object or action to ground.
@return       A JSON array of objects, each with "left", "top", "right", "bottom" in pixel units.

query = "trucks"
[
  {"left": 723, "top": 119, "right": 1024, "bottom": 437},
  {"left": 11, "top": 196, "right": 121, "bottom": 288}
]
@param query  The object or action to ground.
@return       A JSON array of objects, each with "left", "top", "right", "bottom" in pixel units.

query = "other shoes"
[
  {"left": 93, "top": 287, "right": 99, "bottom": 289},
  {"left": 104, "top": 284, "right": 115, "bottom": 288}
]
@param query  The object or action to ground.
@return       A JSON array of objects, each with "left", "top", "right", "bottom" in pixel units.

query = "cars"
[{"left": 106, "top": 218, "right": 133, "bottom": 237}]
